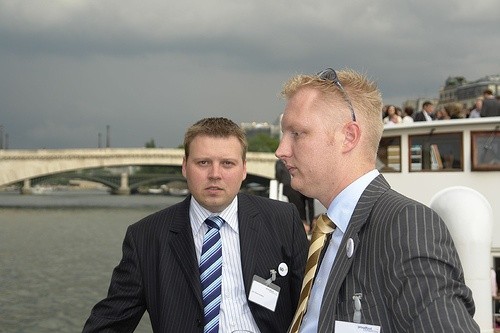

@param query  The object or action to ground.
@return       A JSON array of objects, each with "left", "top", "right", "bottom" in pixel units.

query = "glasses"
[{"left": 317, "top": 66, "right": 357, "bottom": 121}]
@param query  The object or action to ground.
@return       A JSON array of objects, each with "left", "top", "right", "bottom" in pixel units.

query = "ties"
[
  {"left": 199, "top": 215, "right": 225, "bottom": 333},
  {"left": 289, "top": 213, "right": 336, "bottom": 332}
]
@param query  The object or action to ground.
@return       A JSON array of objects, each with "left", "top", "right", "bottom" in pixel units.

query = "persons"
[
  {"left": 275, "top": 157, "right": 324, "bottom": 244},
  {"left": 80, "top": 117, "right": 309, "bottom": 332},
  {"left": 273, "top": 65, "right": 483, "bottom": 333},
  {"left": 378, "top": 84, "right": 500, "bottom": 126}
]
